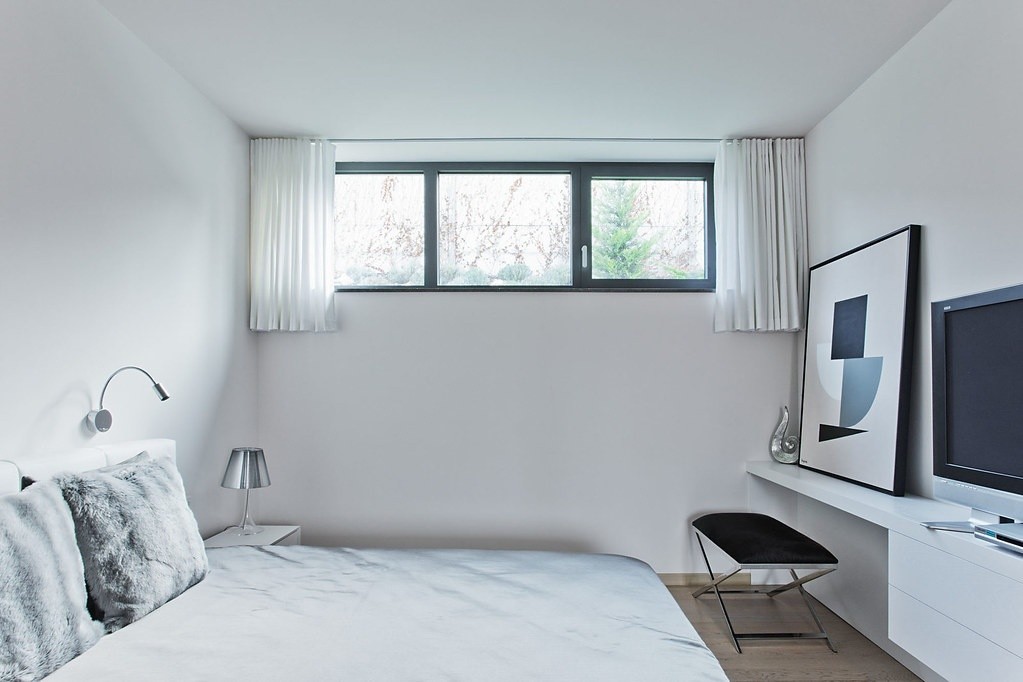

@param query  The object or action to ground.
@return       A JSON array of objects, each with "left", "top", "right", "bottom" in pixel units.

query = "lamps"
[
  {"left": 220, "top": 447, "right": 271, "bottom": 535},
  {"left": 87, "top": 366, "right": 168, "bottom": 434}
]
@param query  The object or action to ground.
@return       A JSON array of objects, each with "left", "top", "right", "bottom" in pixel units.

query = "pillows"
[
  {"left": 58, "top": 451, "right": 211, "bottom": 633},
  {"left": 0, "top": 476, "right": 108, "bottom": 682}
]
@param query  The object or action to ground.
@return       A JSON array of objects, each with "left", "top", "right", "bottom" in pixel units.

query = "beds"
[{"left": 0, "top": 439, "right": 733, "bottom": 682}]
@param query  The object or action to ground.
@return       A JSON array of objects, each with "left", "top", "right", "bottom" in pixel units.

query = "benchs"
[{"left": 691, "top": 511, "right": 838, "bottom": 654}]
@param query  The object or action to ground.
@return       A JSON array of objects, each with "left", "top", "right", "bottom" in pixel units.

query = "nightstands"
[{"left": 203, "top": 523, "right": 302, "bottom": 549}]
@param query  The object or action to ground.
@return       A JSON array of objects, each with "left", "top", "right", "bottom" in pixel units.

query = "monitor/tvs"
[{"left": 930, "top": 283, "right": 1021, "bottom": 524}]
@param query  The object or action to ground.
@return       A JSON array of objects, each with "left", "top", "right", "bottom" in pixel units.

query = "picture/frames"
[{"left": 797, "top": 222, "right": 923, "bottom": 498}]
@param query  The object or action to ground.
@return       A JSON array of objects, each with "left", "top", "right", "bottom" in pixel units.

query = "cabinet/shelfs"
[{"left": 748, "top": 460, "right": 1023, "bottom": 682}]
